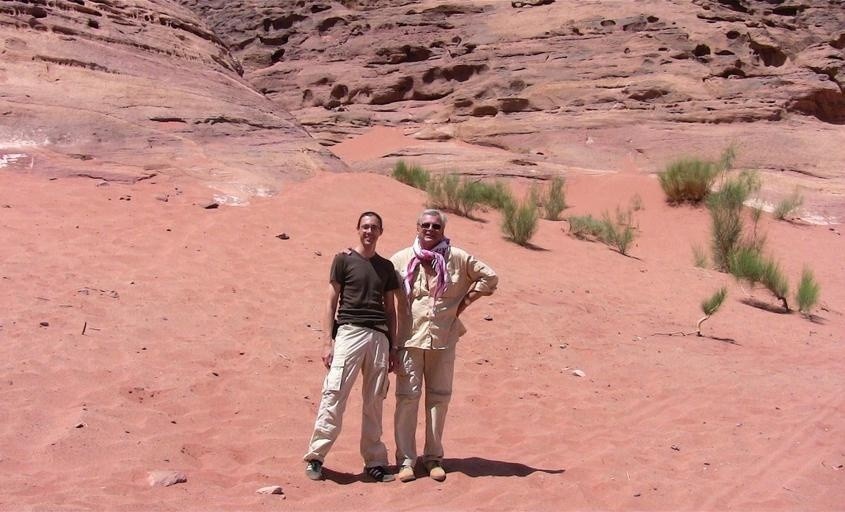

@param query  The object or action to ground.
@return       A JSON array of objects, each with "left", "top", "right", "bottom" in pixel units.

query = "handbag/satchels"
[{"left": 332, "top": 320, "right": 339, "bottom": 340}]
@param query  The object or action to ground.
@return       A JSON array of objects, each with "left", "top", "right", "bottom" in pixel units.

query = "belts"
[{"left": 340, "top": 320, "right": 385, "bottom": 326}]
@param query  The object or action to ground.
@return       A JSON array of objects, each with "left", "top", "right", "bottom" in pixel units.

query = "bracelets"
[
  {"left": 465, "top": 296, "right": 471, "bottom": 306},
  {"left": 391, "top": 344, "right": 399, "bottom": 351}
]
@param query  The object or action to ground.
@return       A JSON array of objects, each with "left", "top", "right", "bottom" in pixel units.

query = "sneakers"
[
  {"left": 366, "top": 465, "right": 416, "bottom": 481},
  {"left": 305, "top": 460, "right": 324, "bottom": 480},
  {"left": 423, "top": 460, "right": 446, "bottom": 481}
]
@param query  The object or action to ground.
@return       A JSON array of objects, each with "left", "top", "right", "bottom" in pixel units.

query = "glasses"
[{"left": 417, "top": 223, "right": 441, "bottom": 229}]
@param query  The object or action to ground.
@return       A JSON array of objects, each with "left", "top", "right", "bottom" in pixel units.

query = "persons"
[
  {"left": 302, "top": 211, "right": 401, "bottom": 483},
  {"left": 334, "top": 208, "right": 498, "bottom": 482}
]
